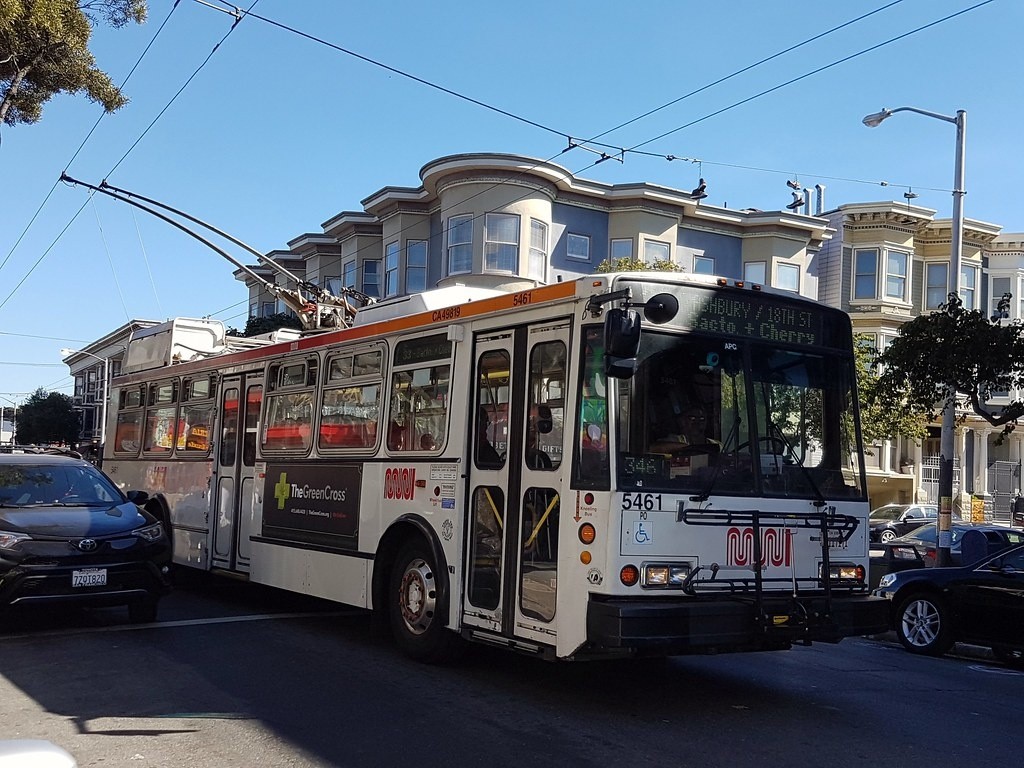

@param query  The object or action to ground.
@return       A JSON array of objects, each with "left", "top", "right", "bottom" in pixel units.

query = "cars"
[
  {"left": 869, "top": 504, "right": 1024, "bottom": 658},
  {"left": 0, "top": 447, "right": 172, "bottom": 623}
]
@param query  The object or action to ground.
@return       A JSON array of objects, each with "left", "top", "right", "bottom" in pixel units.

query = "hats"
[{"left": 373, "top": 421, "right": 407, "bottom": 437}]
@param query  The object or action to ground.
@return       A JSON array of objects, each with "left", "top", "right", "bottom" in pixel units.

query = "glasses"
[
  {"left": 685, "top": 414, "right": 706, "bottom": 422},
  {"left": 425, "top": 442, "right": 435, "bottom": 446},
  {"left": 480, "top": 421, "right": 491, "bottom": 426}
]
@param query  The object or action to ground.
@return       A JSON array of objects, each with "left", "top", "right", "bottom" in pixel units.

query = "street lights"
[
  {"left": 61, "top": 348, "right": 108, "bottom": 447},
  {"left": 863, "top": 108, "right": 967, "bottom": 568}
]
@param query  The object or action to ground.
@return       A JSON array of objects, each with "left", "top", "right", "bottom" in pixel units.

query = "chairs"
[{"left": 116, "top": 421, "right": 211, "bottom": 454}]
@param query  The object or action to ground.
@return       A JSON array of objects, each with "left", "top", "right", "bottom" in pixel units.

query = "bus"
[{"left": 99, "top": 271, "right": 869, "bottom": 662}]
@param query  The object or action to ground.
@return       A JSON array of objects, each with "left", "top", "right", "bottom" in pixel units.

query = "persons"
[
  {"left": 648, "top": 404, "right": 724, "bottom": 460},
  {"left": 478, "top": 408, "right": 499, "bottom": 461},
  {"left": 420, "top": 434, "right": 436, "bottom": 450},
  {"left": 373, "top": 418, "right": 406, "bottom": 451}
]
